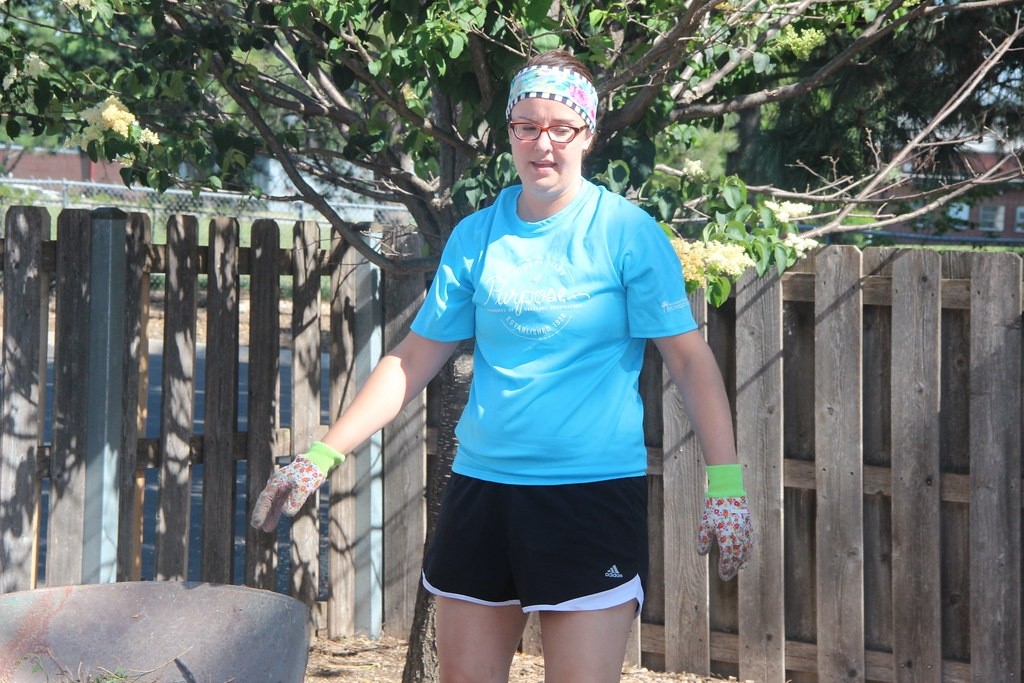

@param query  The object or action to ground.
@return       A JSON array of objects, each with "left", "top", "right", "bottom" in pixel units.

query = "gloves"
[
  {"left": 697, "top": 464, "right": 755, "bottom": 579},
  {"left": 252, "top": 441, "right": 346, "bottom": 532}
]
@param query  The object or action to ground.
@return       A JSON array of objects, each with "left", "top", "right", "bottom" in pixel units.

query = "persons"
[{"left": 247, "top": 52, "right": 751, "bottom": 683}]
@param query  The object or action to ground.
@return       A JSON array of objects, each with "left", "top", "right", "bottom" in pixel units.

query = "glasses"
[{"left": 509, "top": 120, "right": 589, "bottom": 143}]
